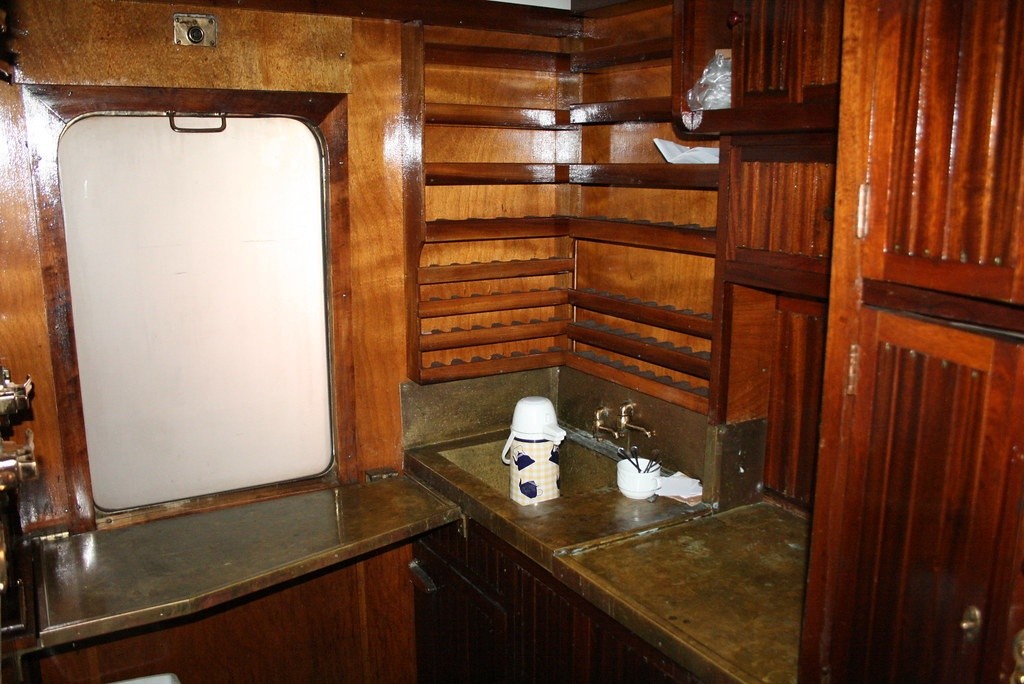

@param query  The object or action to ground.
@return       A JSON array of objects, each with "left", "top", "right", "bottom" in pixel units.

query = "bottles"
[{"left": 502, "top": 396, "right": 566, "bottom": 506}]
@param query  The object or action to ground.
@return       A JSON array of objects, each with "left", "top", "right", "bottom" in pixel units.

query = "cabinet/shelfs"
[{"left": 417, "top": 0, "right": 1024, "bottom": 684}]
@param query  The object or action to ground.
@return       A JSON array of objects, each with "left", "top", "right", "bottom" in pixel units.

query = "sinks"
[{"left": 405, "top": 430, "right": 620, "bottom": 508}]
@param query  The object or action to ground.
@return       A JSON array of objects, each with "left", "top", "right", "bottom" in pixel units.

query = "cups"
[{"left": 616, "top": 458, "right": 660, "bottom": 500}]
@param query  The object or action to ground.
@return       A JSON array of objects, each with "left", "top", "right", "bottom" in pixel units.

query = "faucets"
[
  {"left": 616, "top": 401, "right": 652, "bottom": 440},
  {"left": 592, "top": 406, "right": 620, "bottom": 440}
]
